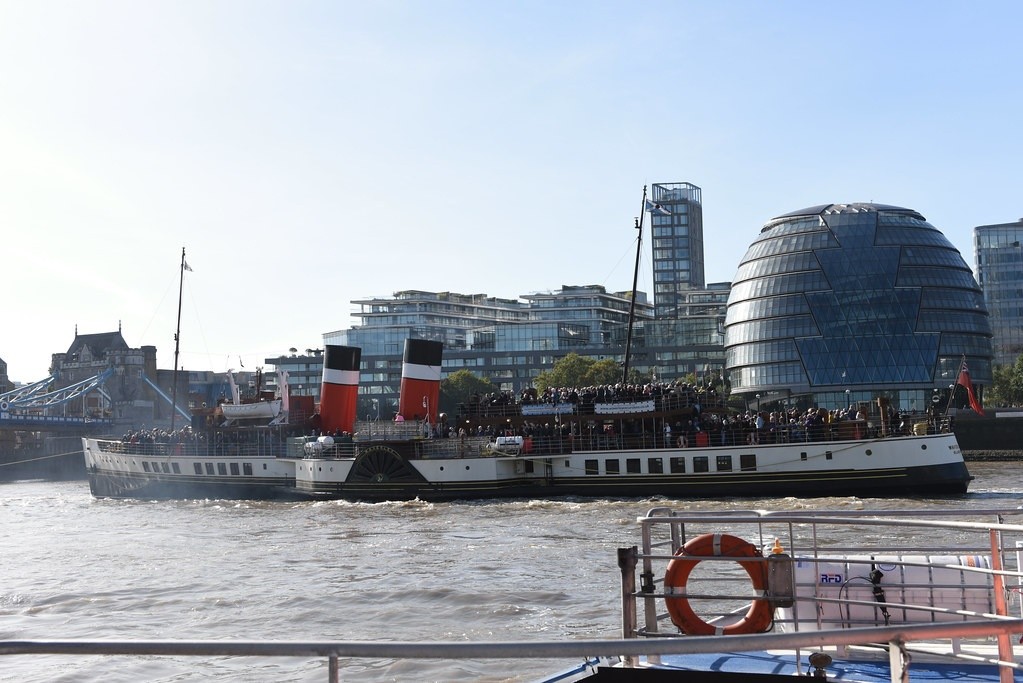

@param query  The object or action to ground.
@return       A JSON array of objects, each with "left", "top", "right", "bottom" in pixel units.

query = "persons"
[{"left": 121, "top": 382, "right": 868, "bottom": 456}]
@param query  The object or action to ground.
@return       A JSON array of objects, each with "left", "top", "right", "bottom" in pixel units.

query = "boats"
[{"left": 219, "top": 366, "right": 282, "bottom": 423}]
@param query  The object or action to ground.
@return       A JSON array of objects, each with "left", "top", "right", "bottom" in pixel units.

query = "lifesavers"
[{"left": 663, "top": 535, "right": 776, "bottom": 636}]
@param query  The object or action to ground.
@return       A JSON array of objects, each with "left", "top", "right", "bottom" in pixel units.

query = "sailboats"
[{"left": 80, "top": 185, "right": 979, "bottom": 504}]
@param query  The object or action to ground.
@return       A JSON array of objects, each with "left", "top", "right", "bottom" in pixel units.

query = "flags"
[{"left": 957, "top": 356, "right": 985, "bottom": 416}]
